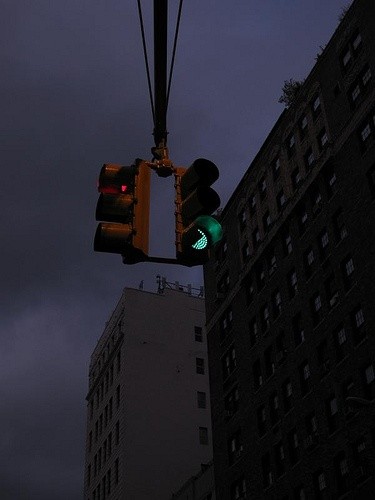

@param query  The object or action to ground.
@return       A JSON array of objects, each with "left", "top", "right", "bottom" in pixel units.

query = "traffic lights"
[
  {"left": 93, "top": 156, "right": 151, "bottom": 265},
  {"left": 174, "top": 156, "right": 225, "bottom": 267}
]
